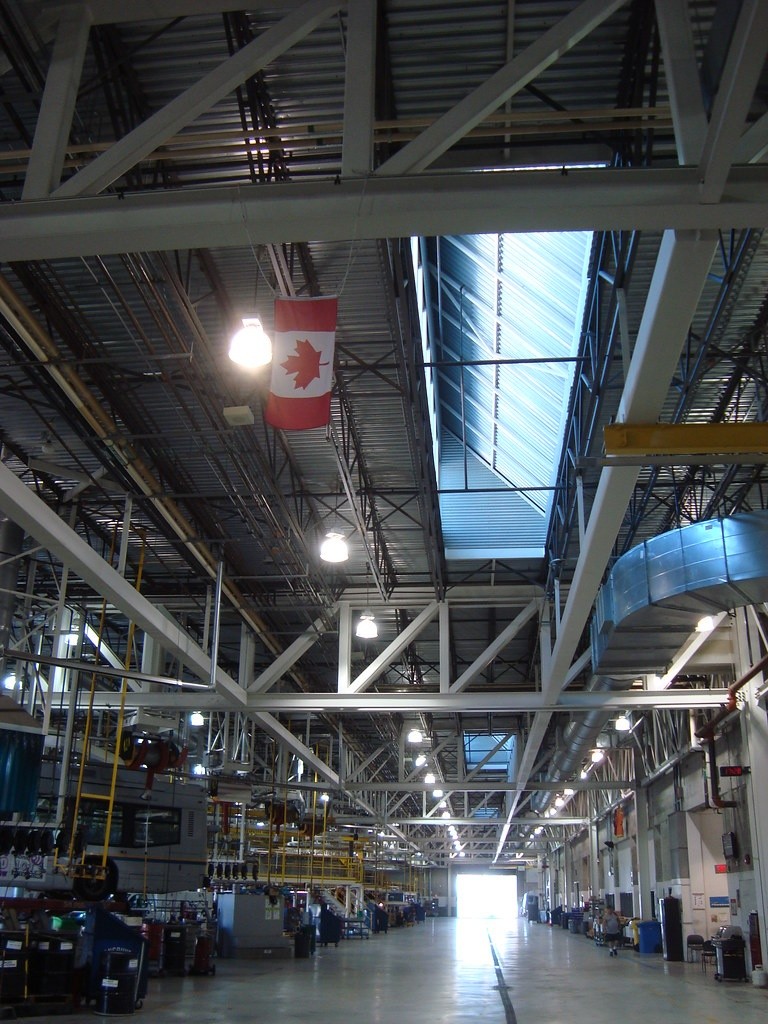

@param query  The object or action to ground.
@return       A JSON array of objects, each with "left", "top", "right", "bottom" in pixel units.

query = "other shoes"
[
  {"left": 609, "top": 952, "right": 613, "bottom": 957},
  {"left": 613, "top": 949, "right": 617, "bottom": 955}
]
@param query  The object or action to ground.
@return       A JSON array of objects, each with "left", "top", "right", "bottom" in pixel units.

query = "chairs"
[
  {"left": 700, "top": 940, "right": 718, "bottom": 972},
  {"left": 686, "top": 934, "right": 704, "bottom": 963}
]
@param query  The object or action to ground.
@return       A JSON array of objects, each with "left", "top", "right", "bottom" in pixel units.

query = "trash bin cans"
[
  {"left": 403, "top": 905, "right": 426, "bottom": 923},
  {"left": 293, "top": 923, "right": 316, "bottom": 959},
  {"left": 635, "top": 920, "right": 661, "bottom": 954},
  {"left": 711, "top": 925, "right": 747, "bottom": 982},
  {"left": 561, "top": 915, "right": 589, "bottom": 935},
  {"left": 630, "top": 918, "right": 644, "bottom": 951}
]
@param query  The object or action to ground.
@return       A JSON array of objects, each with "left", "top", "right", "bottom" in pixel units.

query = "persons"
[{"left": 602, "top": 905, "right": 621, "bottom": 958}]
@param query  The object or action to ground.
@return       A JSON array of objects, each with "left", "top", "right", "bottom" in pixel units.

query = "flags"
[{"left": 263, "top": 292, "right": 338, "bottom": 429}]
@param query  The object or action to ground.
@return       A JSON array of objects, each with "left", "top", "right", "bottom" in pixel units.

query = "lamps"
[
  {"left": 229, "top": 244, "right": 272, "bottom": 373},
  {"left": 319, "top": 471, "right": 348, "bottom": 565},
  {"left": 190, "top": 710, "right": 205, "bottom": 726},
  {"left": 406, "top": 714, "right": 631, "bottom": 861},
  {"left": 64, "top": 618, "right": 82, "bottom": 645},
  {"left": 355, "top": 572, "right": 379, "bottom": 640}
]
